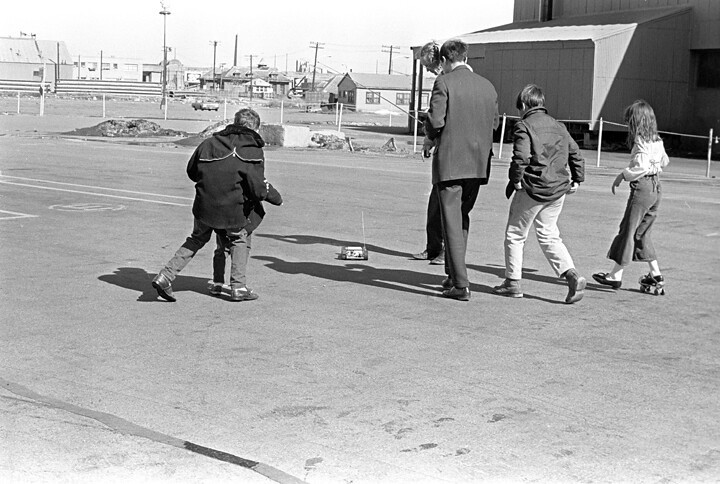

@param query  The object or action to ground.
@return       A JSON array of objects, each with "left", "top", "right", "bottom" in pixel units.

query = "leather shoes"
[
  {"left": 231, "top": 286, "right": 259, "bottom": 300},
  {"left": 442, "top": 276, "right": 453, "bottom": 290},
  {"left": 151, "top": 273, "right": 176, "bottom": 302},
  {"left": 443, "top": 284, "right": 471, "bottom": 301}
]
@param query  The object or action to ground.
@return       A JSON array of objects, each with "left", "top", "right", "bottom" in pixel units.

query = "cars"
[
  {"left": 341, "top": 246, "right": 368, "bottom": 260},
  {"left": 191, "top": 98, "right": 220, "bottom": 111}
]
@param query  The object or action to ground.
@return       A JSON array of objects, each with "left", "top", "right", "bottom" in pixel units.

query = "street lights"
[{"left": 159, "top": 7, "right": 170, "bottom": 108}]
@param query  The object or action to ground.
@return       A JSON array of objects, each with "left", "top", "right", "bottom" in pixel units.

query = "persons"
[
  {"left": 152, "top": 109, "right": 283, "bottom": 301},
  {"left": 490, "top": 85, "right": 587, "bottom": 304},
  {"left": 415, "top": 38, "right": 500, "bottom": 300},
  {"left": 592, "top": 99, "right": 669, "bottom": 295}
]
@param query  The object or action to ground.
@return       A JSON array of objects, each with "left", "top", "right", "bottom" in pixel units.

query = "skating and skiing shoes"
[{"left": 638, "top": 273, "right": 665, "bottom": 295}]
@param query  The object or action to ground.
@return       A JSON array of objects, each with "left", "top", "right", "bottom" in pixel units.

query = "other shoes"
[
  {"left": 565, "top": 269, "right": 585, "bottom": 304},
  {"left": 431, "top": 248, "right": 446, "bottom": 264},
  {"left": 492, "top": 279, "right": 523, "bottom": 298},
  {"left": 413, "top": 250, "right": 428, "bottom": 259},
  {"left": 209, "top": 284, "right": 222, "bottom": 296},
  {"left": 592, "top": 272, "right": 623, "bottom": 290}
]
